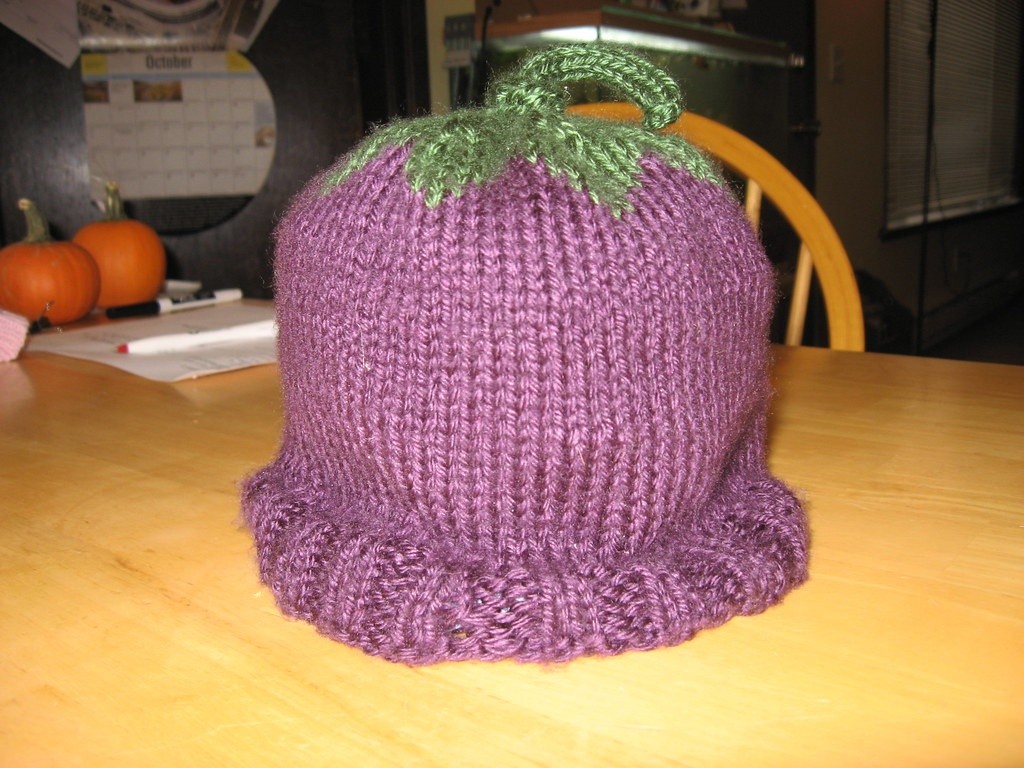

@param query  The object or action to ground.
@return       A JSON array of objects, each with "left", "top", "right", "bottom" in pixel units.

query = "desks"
[{"left": 0, "top": 306, "right": 1024, "bottom": 768}]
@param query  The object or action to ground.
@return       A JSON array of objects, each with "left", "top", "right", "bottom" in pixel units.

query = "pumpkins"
[
  {"left": 74, "top": 182, "right": 167, "bottom": 308},
  {"left": 0, "top": 199, "right": 101, "bottom": 326}
]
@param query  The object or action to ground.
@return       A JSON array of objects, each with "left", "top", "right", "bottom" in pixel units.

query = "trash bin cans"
[{"left": 769, "top": 268, "right": 913, "bottom": 354}]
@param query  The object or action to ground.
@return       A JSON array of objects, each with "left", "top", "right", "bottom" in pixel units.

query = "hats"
[{"left": 245, "top": 43, "right": 813, "bottom": 668}]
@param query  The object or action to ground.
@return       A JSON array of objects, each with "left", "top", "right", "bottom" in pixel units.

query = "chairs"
[{"left": 565, "top": 102, "right": 863, "bottom": 352}]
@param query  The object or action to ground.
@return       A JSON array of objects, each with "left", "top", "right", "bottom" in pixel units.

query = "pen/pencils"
[
  {"left": 105, "top": 288, "right": 243, "bottom": 320},
  {"left": 117, "top": 319, "right": 278, "bottom": 355}
]
